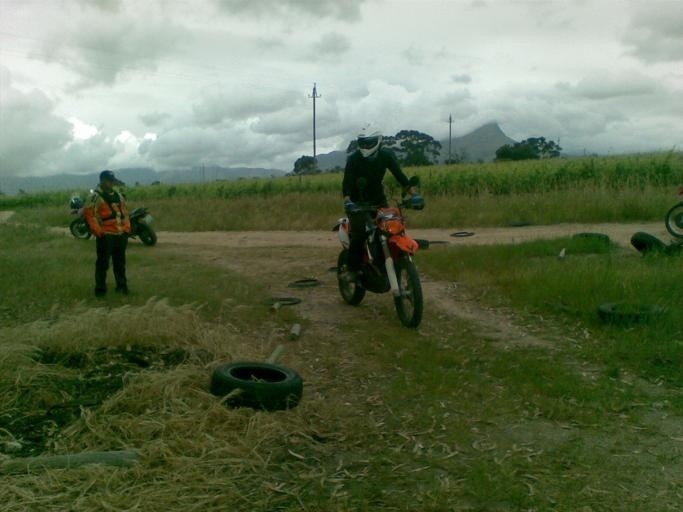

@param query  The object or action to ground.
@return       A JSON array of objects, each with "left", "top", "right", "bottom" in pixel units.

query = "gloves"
[
  {"left": 409, "top": 190, "right": 425, "bottom": 210},
  {"left": 344, "top": 201, "right": 357, "bottom": 214}
]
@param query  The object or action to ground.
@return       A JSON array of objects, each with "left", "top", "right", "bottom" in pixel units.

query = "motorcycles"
[
  {"left": 332, "top": 176, "right": 424, "bottom": 329},
  {"left": 68, "top": 189, "right": 157, "bottom": 245},
  {"left": 665, "top": 184, "right": 683, "bottom": 239}
]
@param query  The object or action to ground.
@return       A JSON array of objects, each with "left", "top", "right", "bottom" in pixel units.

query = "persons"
[
  {"left": 342, "top": 122, "right": 416, "bottom": 283},
  {"left": 84, "top": 171, "right": 131, "bottom": 297}
]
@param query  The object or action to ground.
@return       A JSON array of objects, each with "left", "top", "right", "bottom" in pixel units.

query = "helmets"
[{"left": 358, "top": 121, "right": 383, "bottom": 158}]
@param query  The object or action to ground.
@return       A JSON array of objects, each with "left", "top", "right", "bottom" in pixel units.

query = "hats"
[{"left": 99, "top": 170, "right": 125, "bottom": 185}]
